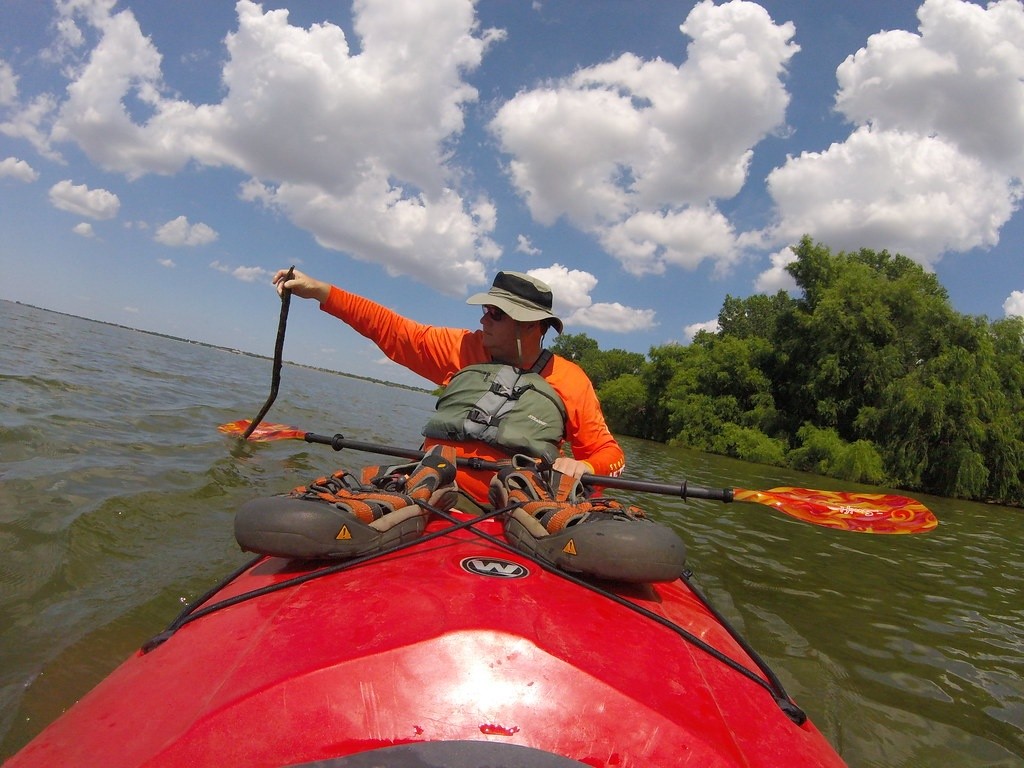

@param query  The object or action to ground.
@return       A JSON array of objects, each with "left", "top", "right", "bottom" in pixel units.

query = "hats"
[{"left": 466, "top": 270, "right": 563, "bottom": 336}]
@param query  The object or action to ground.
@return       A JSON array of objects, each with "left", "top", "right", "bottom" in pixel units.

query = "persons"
[{"left": 272, "top": 268, "right": 628, "bottom": 512}]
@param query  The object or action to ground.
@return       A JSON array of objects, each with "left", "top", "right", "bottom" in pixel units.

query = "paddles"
[{"left": 217, "top": 416, "right": 938, "bottom": 536}]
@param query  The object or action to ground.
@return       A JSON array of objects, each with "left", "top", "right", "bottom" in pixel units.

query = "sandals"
[
  {"left": 233, "top": 445, "right": 459, "bottom": 561},
  {"left": 488, "top": 454, "right": 688, "bottom": 583}
]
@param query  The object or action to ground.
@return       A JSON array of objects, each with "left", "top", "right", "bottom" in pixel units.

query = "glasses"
[{"left": 480, "top": 305, "right": 505, "bottom": 322}]
